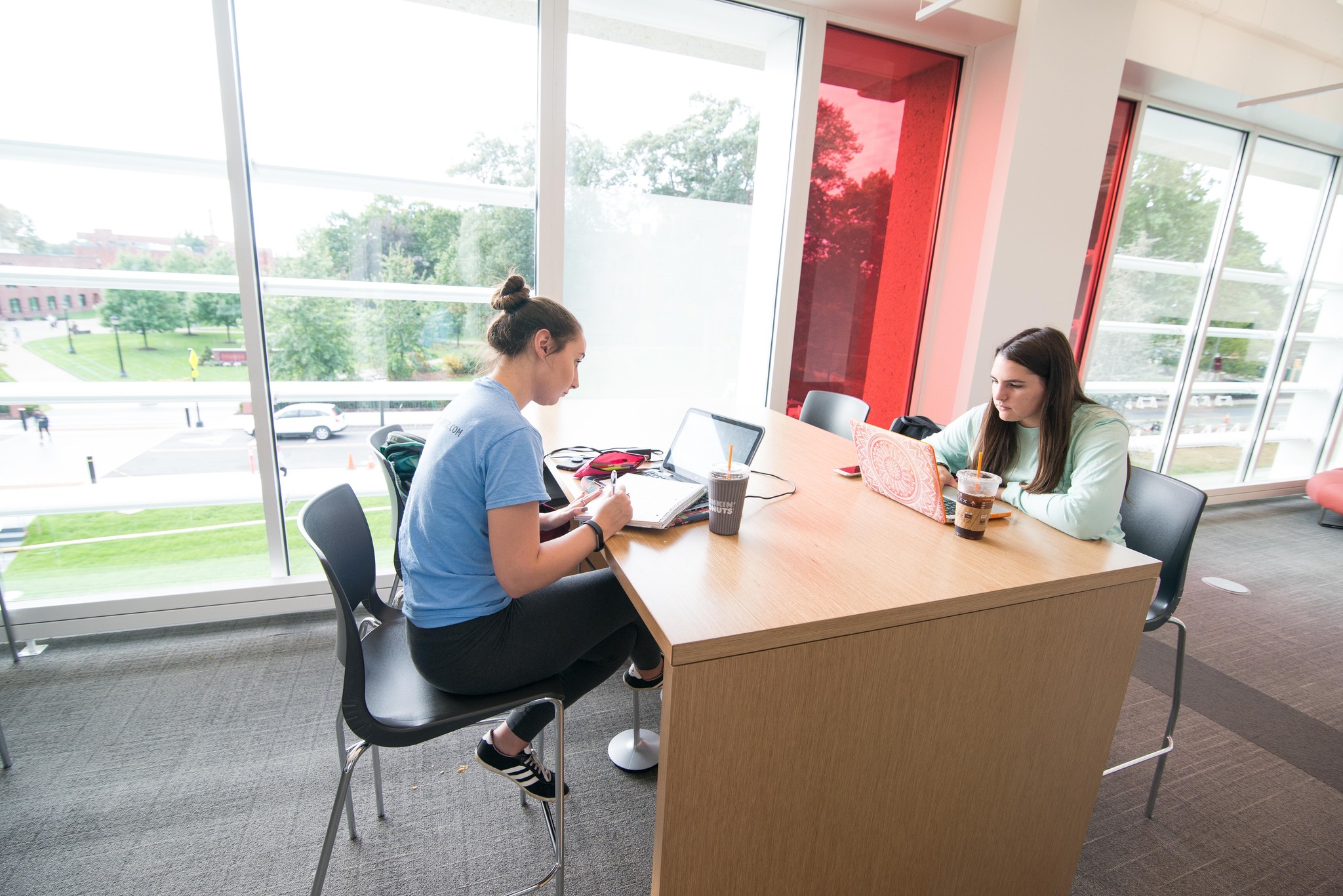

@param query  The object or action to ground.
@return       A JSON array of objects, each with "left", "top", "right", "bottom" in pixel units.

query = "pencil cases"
[{"left": 571, "top": 451, "right": 649, "bottom": 478}]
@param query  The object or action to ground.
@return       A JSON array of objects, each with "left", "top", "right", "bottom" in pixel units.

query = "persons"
[
  {"left": 398, "top": 274, "right": 663, "bottom": 802},
  {"left": 920, "top": 327, "right": 1131, "bottom": 548},
  {"left": 32, "top": 407, "right": 51, "bottom": 440}
]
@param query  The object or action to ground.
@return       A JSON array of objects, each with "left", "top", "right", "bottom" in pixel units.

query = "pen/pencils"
[
  {"left": 594, "top": 458, "right": 638, "bottom": 471},
  {"left": 610, "top": 470, "right": 618, "bottom": 497}
]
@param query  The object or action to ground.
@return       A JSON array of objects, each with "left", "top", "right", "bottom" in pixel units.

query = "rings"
[
  {"left": 626, "top": 494, "right": 630, "bottom": 499},
  {"left": 580, "top": 499, "right": 586, "bottom": 505}
]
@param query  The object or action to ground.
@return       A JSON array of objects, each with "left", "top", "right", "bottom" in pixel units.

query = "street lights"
[
  {"left": 109, "top": 315, "right": 128, "bottom": 377},
  {"left": 374, "top": 375, "right": 387, "bottom": 427},
  {"left": 61, "top": 301, "right": 76, "bottom": 353}
]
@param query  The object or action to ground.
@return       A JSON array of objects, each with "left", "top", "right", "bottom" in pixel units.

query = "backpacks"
[{"left": 891, "top": 410, "right": 940, "bottom": 441}]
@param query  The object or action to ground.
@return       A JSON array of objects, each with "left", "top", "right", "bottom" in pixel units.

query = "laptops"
[
  {"left": 592, "top": 407, "right": 766, "bottom": 515},
  {"left": 850, "top": 418, "right": 1012, "bottom": 524}
]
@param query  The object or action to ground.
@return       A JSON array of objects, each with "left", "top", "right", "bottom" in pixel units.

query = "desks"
[{"left": 541, "top": 407, "right": 1166, "bottom": 896}]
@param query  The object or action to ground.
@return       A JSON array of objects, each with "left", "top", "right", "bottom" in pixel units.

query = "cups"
[
  {"left": 954, "top": 469, "right": 1002, "bottom": 540},
  {"left": 708, "top": 462, "right": 750, "bottom": 536}
]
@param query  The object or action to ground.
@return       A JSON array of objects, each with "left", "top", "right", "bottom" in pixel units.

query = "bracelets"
[{"left": 580, "top": 520, "right": 604, "bottom": 552}]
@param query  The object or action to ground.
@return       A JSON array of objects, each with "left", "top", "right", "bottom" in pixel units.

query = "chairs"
[
  {"left": 799, "top": 390, "right": 871, "bottom": 442},
  {"left": 888, "top": 416, "right": 946, "bottom": 444},
  {"left": 370, "top": 426, "right": 434, "bottom": 598},
  {"left": 300, "top": 485, "right": 567, "bottom": 896},
  {"left": 1114, "top": 467, "right": 1210, "bottom": 818}
]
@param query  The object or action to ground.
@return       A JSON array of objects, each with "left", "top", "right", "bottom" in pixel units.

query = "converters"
[{"left": 626, "top": 449, "right": 652, "bottom": 460}]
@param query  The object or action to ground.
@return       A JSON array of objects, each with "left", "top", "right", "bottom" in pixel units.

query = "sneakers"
[
  {"left": 475, "top": 729, "right": 570, "bottom": 803},
  {"left": 623, "top": 652, "right": 666, "bottom": 690}
]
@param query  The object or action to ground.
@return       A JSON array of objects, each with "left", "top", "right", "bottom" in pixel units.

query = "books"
[{"left": 574, "top": 473, "right": 708, "bottom": 529}]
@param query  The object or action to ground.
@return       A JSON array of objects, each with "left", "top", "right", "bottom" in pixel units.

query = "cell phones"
[
  {"left": 556, "top": 453, "right": 594, "bottom": 472},
  {"left": 833, "top": 465, "right": 861, "bottom": 477}
]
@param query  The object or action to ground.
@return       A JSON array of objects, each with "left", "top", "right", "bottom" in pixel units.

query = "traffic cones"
[
  {"left": 1224, "top": 414, "right": 1230, "bottom": 424},
  {"left": 367, "top": 451, "right": 374, "bottom": 469},
  {"left": 347, "top": 453, "right": 355, "bottom": 468}
]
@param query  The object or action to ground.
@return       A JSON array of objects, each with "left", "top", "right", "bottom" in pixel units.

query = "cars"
[{"left": 245, "top": 403, "right": 348, "bottom": 441}]
